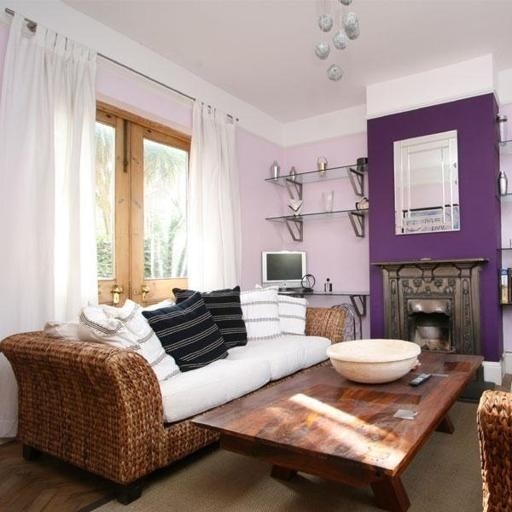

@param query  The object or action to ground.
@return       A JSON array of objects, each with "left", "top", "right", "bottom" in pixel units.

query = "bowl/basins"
[{"left": 326, "top": 337, "right": 421, "bottom": 383}]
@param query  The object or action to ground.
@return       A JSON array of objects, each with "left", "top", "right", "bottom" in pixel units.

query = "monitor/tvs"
[{"left": 261, "top": 251, "right": 307, "bottom": 293}]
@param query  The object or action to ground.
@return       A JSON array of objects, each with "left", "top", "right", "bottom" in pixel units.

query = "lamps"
[{"left": 315, "top": 1, "right": 358, "bottom": 81}]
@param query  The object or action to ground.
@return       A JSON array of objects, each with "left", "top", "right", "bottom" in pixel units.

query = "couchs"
[{"left": 2, "top": 295, "right": 345, "bottom": 502}]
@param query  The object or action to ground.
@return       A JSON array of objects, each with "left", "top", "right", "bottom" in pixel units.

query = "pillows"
[{"left": 81, "top": 287, "right": 307, "bottom": 381}]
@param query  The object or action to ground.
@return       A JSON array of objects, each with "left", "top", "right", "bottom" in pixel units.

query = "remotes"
[{"left": 409, "top": 372, "right": 432, "bottom": 386}]
